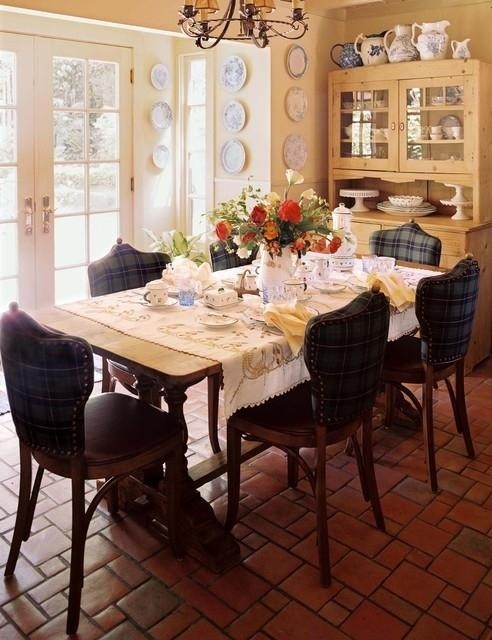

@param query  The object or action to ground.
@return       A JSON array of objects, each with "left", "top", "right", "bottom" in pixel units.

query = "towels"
[
  {"left": 264, "top": 304, "right": 314, "bottom": 356},
  {"left": 369, "top": 273, "right": 415, "bottom": 311}
]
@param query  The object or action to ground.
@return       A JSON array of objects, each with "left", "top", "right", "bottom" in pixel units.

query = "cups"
[
  {"left": 236, "top": 204, "right": 395, "bottom": 304},
  {"left": 342, "top": 91, "right": 463, "bottom": 161},
  {"left": 144, "top": 286, "right": 169, "bottom": 304},
  {"left": 178, "top": 289, "right": 196, "bottom": 306}
]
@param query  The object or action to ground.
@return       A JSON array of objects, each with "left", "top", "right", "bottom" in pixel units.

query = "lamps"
[{"left": 177, "top": 0, "right": 310, "bottom": 49}]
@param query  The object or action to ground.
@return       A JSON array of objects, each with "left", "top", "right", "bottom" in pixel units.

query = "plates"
[
  {"left": 375, "top": 195, "right": 437, "bottom": 217},
  {"left": 200, "top": 299, "right": 244, "bottom": 310},
  {"left": 141, "top": 297, "right": 177, "bottom": 309},
  {"left": 304, "top": 306, "right": 320, "bottom": 317},
  {"left": 199, "top": 316, "right": 237, "bottom": 328},
  {"left": 149, "top": 45, "right": 309, "bottom": 175},
  {"left": 170, "top": 291, "right": 179, "bottom": 298}
]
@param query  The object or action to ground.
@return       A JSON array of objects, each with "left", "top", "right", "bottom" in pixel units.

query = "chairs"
[
  {"left": 88, "top": 239, "right": 221, "bottom": 472},
  {"left": 347, "top": 252, "right": 480, "bottom": 491},
  {"left": 369, "top": 218, "right": 442, "bottom": 388},
  {"left": 224, "top": 285, "right": 390, "bottom": 588},
  {"left": 0, "top": 301, "right": 184, "bottom": 635},
  {"left": 209, "top": 241, "right": 259, "bottom": 271}
]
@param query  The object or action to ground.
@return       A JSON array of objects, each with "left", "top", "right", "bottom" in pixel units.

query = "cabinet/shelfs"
[
  {"left": 327, "top": 218, "right": 492, "bottom": 376},
  {"left": 328, "top": 59, "right": 492, "bottom": 224}
]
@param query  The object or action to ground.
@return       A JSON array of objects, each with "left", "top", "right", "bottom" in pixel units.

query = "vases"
[{"left": 259, "top": 245, "right": 293, "bottom": 304}]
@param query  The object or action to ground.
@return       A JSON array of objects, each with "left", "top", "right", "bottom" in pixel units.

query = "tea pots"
[{"left": 330, "top": 20, "right": 470, "bottom": 69}]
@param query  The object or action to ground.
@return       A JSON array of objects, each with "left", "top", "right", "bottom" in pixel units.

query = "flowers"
[{"left": 199, "top": 169, "right": 344, "bottom": 259}]
